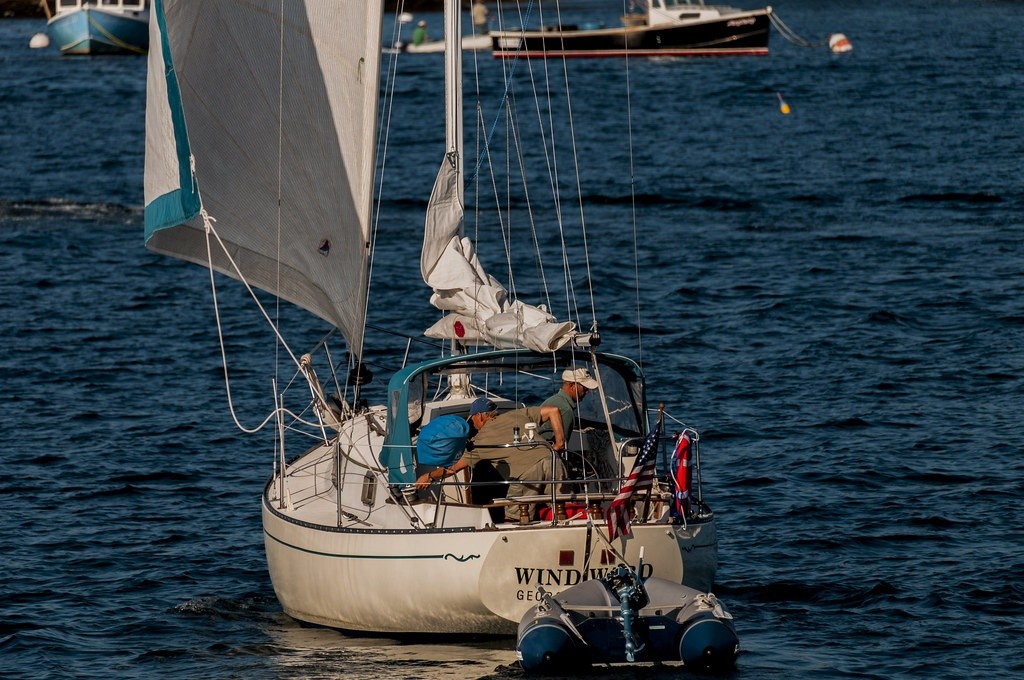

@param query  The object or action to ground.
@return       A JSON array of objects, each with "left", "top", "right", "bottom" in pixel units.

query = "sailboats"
[{"left": 144, "top": 0, "right": 718, "bottom": 635}]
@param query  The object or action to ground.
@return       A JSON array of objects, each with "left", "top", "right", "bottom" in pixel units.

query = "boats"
[
  {"left": 382, "top": 34, "right": 493, "bottom": 54},
  {"left": 518, "top": 546, "right": 739, "bottom": 680},
  {"left": 488, "top": 0, "right": 772, "bottom": 54},
  {"left": 40, "top": 0, "right": 150, "bottom": 55}
]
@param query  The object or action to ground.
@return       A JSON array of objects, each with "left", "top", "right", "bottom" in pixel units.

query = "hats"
[
  {"left": 562, "top": 369, "right": 600, "bottom": 389},
  {"left": 465, "top": 397, "right": 497, "bottom": 422}
]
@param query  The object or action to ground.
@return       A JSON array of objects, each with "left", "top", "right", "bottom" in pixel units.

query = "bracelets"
[{"left": 428, "top": 473, "right": 436, "bottom": 484}]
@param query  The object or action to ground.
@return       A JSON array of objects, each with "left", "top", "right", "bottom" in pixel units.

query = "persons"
[
  {"left": 535, "top": 365, "right": 599, "bottom": 451},
  {"left": 415, "top": 398, "right": 576, "bottom": 523},
  {"left": 470, "top": 0, "right": 490, "bottom": 36},
  {"left": 412, "top": 20, "right": 432, "bottom": 46}
]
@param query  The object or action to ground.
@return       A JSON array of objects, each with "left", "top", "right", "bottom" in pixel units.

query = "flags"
[{"left": 607, "top": 420, "right": 662, "bottom": 545}]
